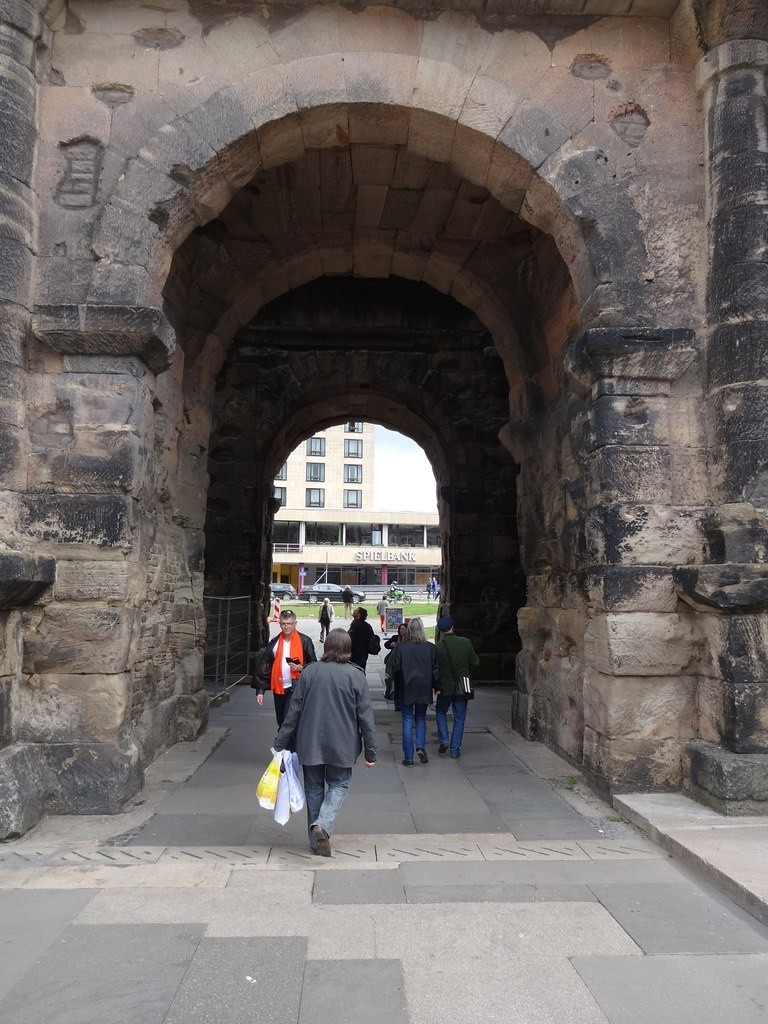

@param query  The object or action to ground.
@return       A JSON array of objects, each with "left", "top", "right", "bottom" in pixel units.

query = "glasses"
[
  {"left": 281, "top": 610, "right": 292, "bottom": 615},
  {"left": 280, "top": 621, "right": 294, "bottom": 627}
]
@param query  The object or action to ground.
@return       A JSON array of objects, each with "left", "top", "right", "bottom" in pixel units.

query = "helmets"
[
  {"left": 345, "top": 585, "right": 351, "bottom": 590},
  {"left": 393, "top": 581, "right": 398, "bottom": 584}
]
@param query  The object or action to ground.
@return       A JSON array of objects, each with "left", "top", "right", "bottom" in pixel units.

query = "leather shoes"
[{"left": 310, "top": 825, "right": 330, "bottom": 856}]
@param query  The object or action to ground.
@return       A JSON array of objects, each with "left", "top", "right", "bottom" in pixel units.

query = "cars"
[
  {"left": 298, "top": 582, "right": 366, "bottom": 604},
  {"left": 270, "top": 583, "right": 296, "bottom": 600}
]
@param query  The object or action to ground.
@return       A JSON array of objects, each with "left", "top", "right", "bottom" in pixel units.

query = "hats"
[{"left": 438, "top": 616, "right": 453, "bottom": 631}]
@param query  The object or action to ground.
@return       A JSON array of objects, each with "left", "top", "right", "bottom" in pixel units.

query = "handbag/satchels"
[
  {"left": 256, "top": 748, "right": 304, "bottom": 826},
  {"left": 385, "top": 676, "right": 395, "bottom": 700},
  {"left": 457, "top": 672, "right": 475, "bottom": 701},
  {"left": 369, "top": 634, "right": 380, "bottom": 655}
]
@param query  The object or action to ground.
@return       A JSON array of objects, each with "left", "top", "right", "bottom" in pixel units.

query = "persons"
[
  {"left": 384, "top": 617, "right": 479, "bottom": 768},
  {"left": 250, "top": 610, "right": 317, "bottom": 733},
  {"left": 318, "top": 597, "right": 334, "bottom": 643},
  {"left": 342, "top": 585, "right": 354, "bottom": 619},
  {"left": 426, "top": 577, "right": 437, "bottom": 599},
  {"left": 274, "top": 629, "right": 378, "bottom": 856},
  {"left": 377, "top": 595, "right": 391, "bottom": 632},
  {"left": 347, "top": 607, "right": 373, "bottom": 674},
  {"left": 390, "top": 581, "right": 399, "bottom": 603}
]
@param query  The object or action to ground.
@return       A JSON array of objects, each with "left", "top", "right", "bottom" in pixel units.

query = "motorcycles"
[{"left": 383, "top": 588, "right": 412, "bottom": 604}]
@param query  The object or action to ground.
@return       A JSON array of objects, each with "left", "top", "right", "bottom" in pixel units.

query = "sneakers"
[
  {"left": 451, "top": 752, "right": 461, "bottom": 758},
  {"left": 438, "top": 743, "right": 448, "bottom": 754},
  {"left": 417, "top": 749, "right": 429, "bottom": 765},
  {"left": 402, "top": 759, "right": 413, "bottom": 766},
  {"left": 319, "top": 639, "right": 324, "bottom": 643}
]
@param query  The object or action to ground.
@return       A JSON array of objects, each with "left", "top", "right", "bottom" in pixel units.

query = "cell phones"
[{"left": 286, "top": 657, "right": 301, "bottom": 665}]
